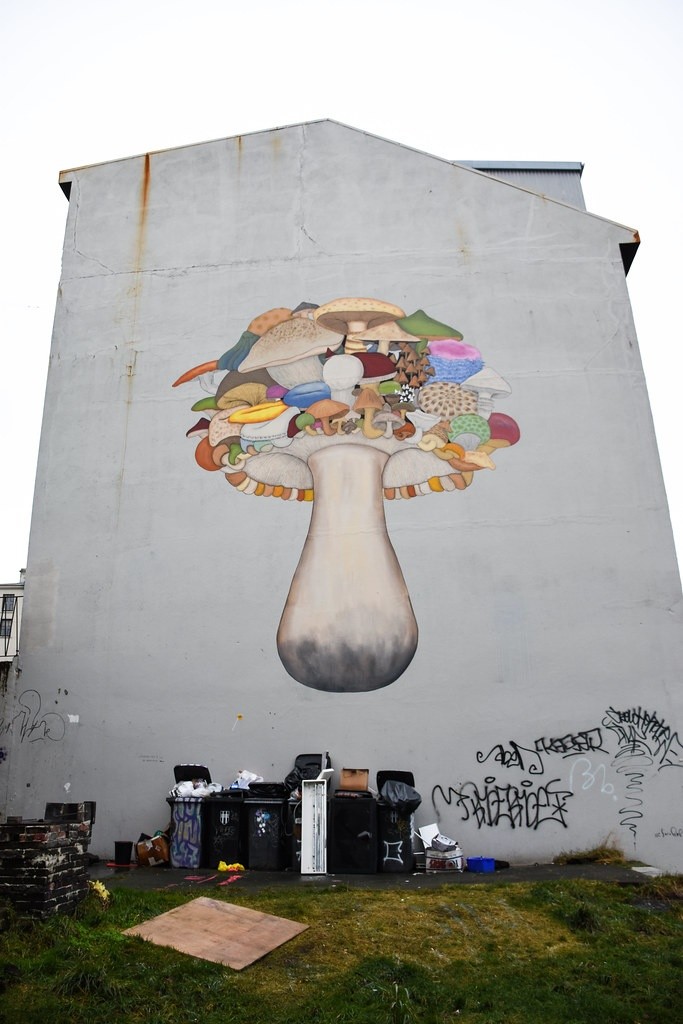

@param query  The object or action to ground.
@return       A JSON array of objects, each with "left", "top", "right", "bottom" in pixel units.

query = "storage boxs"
[
  {"left": 137, "top": 836, "right": 168, "bottom": 869},
  {"left": 466, "top": 856, "right": 495, "bottom": 873},
  {"left": 425, "top": 833, "right": 465, "bottom": 874}
]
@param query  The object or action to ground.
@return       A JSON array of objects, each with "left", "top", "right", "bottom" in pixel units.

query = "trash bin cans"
[
  {"left": 376, "top": 769, "right": 417, "bottom": 876},
  {"left": 287, "top": 753, "right": 332, "bottom": 870},
  {"left": 202, "top": 788, "right": 245, "bottom": 872},
  {"left": 326, "top": 789, "right": 376, "bottom": 875},
  {"left": 165, "top": 764, "right": 211, "bottom": 870},
  {"left": 242, "top": 781, "right": 288, "bottom": 871}
]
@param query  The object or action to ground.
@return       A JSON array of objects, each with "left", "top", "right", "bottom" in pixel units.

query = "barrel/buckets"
[{"left": 114, "top": 840, "right": 133, "bottom": 865}]
